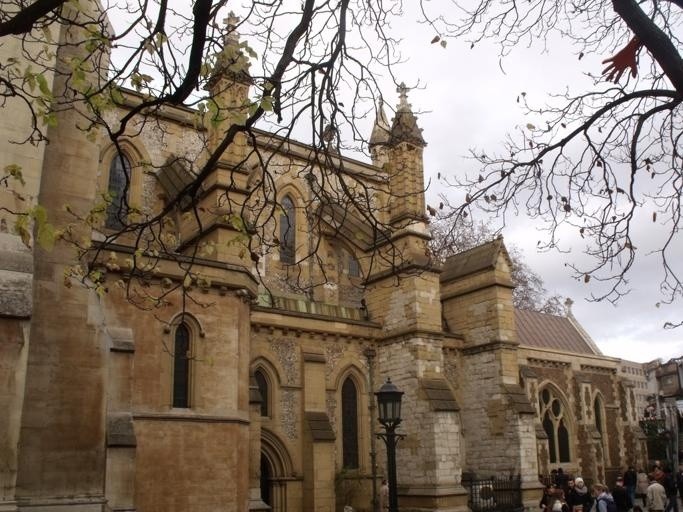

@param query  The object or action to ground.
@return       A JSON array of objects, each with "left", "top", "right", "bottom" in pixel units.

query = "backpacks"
[{"left": 596, "top": 497, "right": 618, "bottom": 511}]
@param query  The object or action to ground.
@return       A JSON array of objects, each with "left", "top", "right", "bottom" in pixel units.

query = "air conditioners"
[{"left": 472, "top": 482, "right": 495, "bottom": 510}]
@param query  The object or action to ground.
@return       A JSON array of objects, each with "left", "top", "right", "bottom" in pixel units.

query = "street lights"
[{"left": 374, "top": 377, "right": 408, "bottom": 510}]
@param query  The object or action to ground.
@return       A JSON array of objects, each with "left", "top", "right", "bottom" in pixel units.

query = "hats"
[{"left": 575, "top": 477, "right": 584, "bottom": 486}]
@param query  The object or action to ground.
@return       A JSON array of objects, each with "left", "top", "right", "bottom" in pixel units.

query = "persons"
[{"left": 537, "top": 461, "right": 667, "bottom": 512}]
